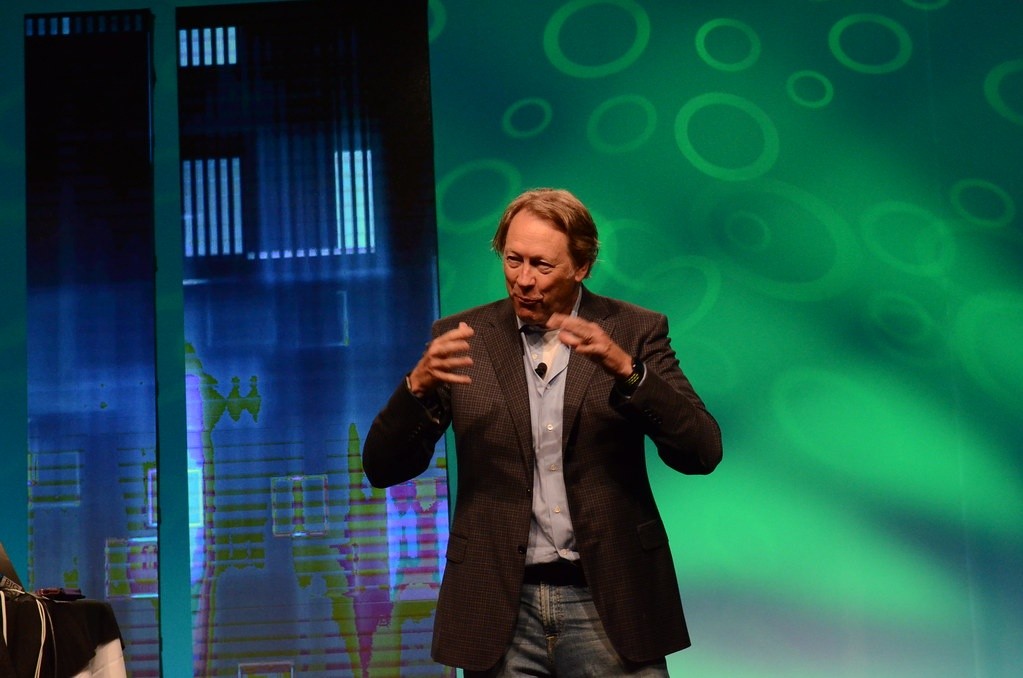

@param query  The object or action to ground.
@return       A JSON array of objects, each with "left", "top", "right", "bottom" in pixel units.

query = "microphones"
[{"left": 535, "top": 362, "right": 547, "bottom": 379}]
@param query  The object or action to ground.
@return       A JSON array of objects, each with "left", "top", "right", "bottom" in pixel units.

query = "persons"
[{"left": 363, "top": 187, "right": 723, "bottom": 678}]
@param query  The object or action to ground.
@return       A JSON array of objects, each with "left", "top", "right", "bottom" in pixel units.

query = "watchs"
[{"left": 618, "top": 353, "right": 644, "bottom": 390}]
